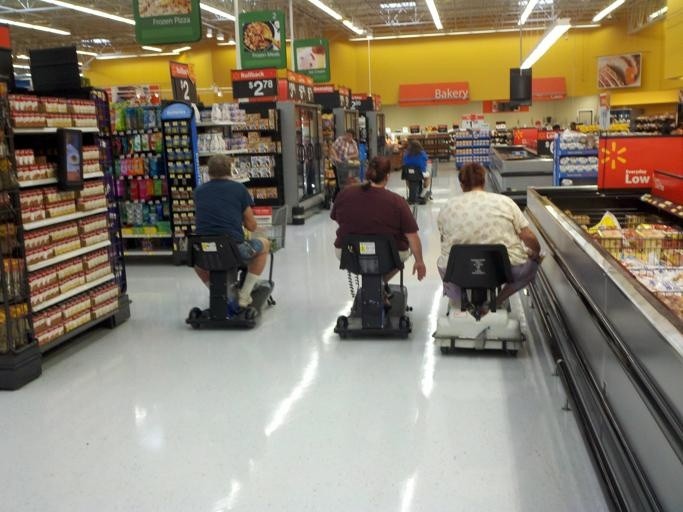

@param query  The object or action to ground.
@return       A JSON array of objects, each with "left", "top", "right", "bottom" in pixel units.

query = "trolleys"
[
  {"left": 404, "top": 164, "right": 433, "bottom": 205},
  {"left": 334, "top": 160, "right": 360, "bottom": 192}
]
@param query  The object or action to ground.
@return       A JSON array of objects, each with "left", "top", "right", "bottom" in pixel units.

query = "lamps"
[
  {"left": 77, "top": 61, "right": 83, "bottom": 70},
  {"left": 649, "top": 4, "right": 668, "bottom": 21},
  {"left": 217, "top": 42, "right": 230, "bottom": 46},
  {"left": 341, "top": 19, "right": 366, "bottom": 37},
  {"left": 141, "top": 45, "right": 164, "bottom": 53},
  {"left": 140, "top": 51, "right": 179, "bottom": 57},
  {"left": 199, "top": 2, "right": 236, "bottom": 24},
  {"left": 96, "top": 52, "right": 138, "bottom": 59},
  {"left": 518, "top": 2, "right": 554, "bottom": 5},
  {"left": 205, "top": 28, "right": 213, "bottom": 39},
  {"left": 425, "top": 3, "right": 444, "bottom": 31},
  {"left": 592, "top": 1, "right": 623, "bottom": 24},
  {"left": 215, "top": 31, "right": 225, "bottom": 41},
  {"left": 347, "top": 21, "right": 600, "bottom": 42},
  {"left": 311, "top": 0, "right": 344, "bottom": 22},
  {"left": 516, "top": 0, "right": 539, "bottom": 26},
  {"left": 521, "top": 7, "right": 573, "bottom": 73},
  {"left": 13, "top": 54, "right": 31, "bottom": 60},
  {"left": 83, "top": 37, "right": 112, "bottom": 46},
  {"left": 1, "top": 17, "right": 73, "bottom": 36},
  {"left": 75, "top": 47, "right": 98, "bottom": 57},
  {"left": 172, "top": 45, "right": 192, "bottom": 54},
  {"left": 228, "top": 35, "right": 236, "bottom": 46},
  {"left": 45, "top": 1, "right": 137, "bottom": 26},
  {"left": 378, "top": 1, "right": 417, "bottom": 9},
  {"left": 13, "top": 64, "right": 31, "bottom": 77}
]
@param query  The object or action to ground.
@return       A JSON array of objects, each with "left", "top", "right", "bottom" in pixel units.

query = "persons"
[
  {"left": 192, "top": 152, "right": 269, "bottom": 308},
  {"left": 569, "top": 121, "right": 576, "bottom": 130},
  {"left": 400, "top": 139, "right": 431, "bottom": 188},
  {"left": 324, "top": 123, "right": 339, "bottom": 161},
  {"left": 327, "top": 155, "right": 427, "bottom": 309},
  {"left": 329, "top": 126, "right": 358, "bottom": 203},
  {"left": 660, "top": 122, "right": 670, "bottom": 135},
  {"left": 551, "top": 124, "right": 560, "bottom": 130},
  {"left": 435, "top": 162, "right": 544, "bottom": 314}
]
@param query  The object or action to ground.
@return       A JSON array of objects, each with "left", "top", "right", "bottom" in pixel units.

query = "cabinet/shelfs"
[
  {"left": 98, "top": 83, "right": 283, "bottom": 267},
  {"left": 279, "top": 85, "right": 383, "bottom": 228},
  {"left": 385, "top": 107, "right": 676, "bottom": 189},
  {"left": 1, "top": 84, "right": 131, "bottom": 391}
]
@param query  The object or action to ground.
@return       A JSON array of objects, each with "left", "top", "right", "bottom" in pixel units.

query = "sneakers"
[
  {"left": 384, "top": 292, "right": 391, "bottom": 307},
  {"left": 238, "top": 293, "right": 255, "bottom": 308}
]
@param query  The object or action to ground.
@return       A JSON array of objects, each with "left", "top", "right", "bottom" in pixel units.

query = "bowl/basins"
[{"left": 242, "top": 21, "right": 275, "bottom": 50}]
